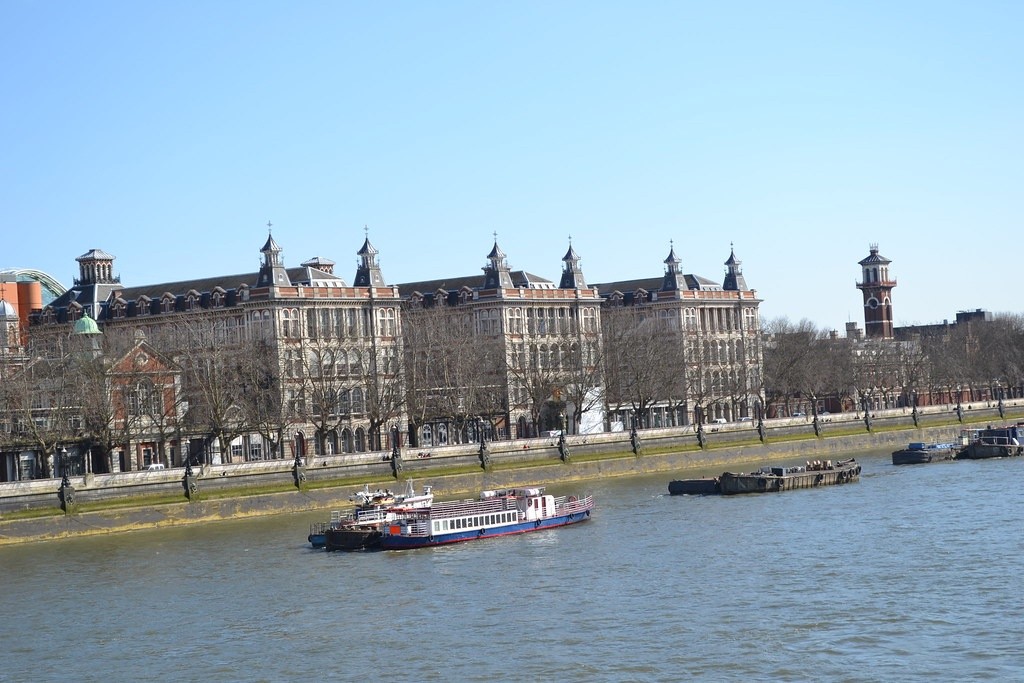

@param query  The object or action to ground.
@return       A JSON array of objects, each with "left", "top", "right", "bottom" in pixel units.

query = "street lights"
[
  {"left": 479, "top": 417, "right": 492, "bottom": 470},
  {"left": 393, "top": 421, "right": 403, "bottom": 480},
  {"left": 184, "top": 438, "right": 200, "bottom": 502},
  {"left": 696, "top": 402, "right": 707, "bottom": 449},
  {"left": 558, "top": 412, "right": 571, "bottom": 460},
  {"left": 755, "top": 398, "right": 768, "bottom": 443},
  {"left": 59, "top": 445, "right": 77, "bottom": 515},
  {"left": 956, "top": 385, "right": 964, "bottom": 423},
  {"left": 631, "top": 407, "right": 642, "bottom": 453},
  {"left": 863, "top": 390, "right": 872, "bottom": 432},
  {"left": 294, "top": 431, "right": 306, "bottom": 491},
  {"left": 910, "top": 388, "right": 920, "bottom": 426},
  {"left": 812, "top": 396, "right": 822, "bottom": 436},
  {"left": 997, "top": 383, "right": 1006, "bottom": 419}
]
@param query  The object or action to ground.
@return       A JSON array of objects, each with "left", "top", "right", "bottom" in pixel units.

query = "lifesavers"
[
  {"left": 1018, "top": 446, "right": 1024, "bottom": 454},
  {"left": 840, "top": 471, "right": 847, "bottom": 479},
  {"left": 481, "top": 528, "right": 486, "bottom": 535},
  {"left": 586, "top": 510, "right": 591, "bottom": 516},
  {"left": 845, "top": 474, "right": 850, "bottom": 483},
  {"left": 776, "top": 478, "right": 785, "bottom": 487},
  {"left": 849, "top": 468, "right": 856, "bottom": 477},
  {"left": 757, "top": 477, "right": 768, "bottom": 487},
  {"left": 856, "top": 466, "right": 862, "bottom": 475},
  {"left": 537, "top": 519, "right": 542, "bottom": 525},
  {"left": 1007, "top": 448, "right": 1013, "bottom": 456},
  {"left": 818, "top": 474, "right": 824, "bottom": 482},
  {"left": 568, "top": 495, "right": 577, "bottom": 502},
  {"left": 570, "top": 513, "right": 575, "bottom": 519},
  {"left": 428, "top": 534, "right": 434, "bottom": 543}
]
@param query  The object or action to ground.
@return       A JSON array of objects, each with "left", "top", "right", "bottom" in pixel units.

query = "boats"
[
  {"left": 305, "top": 477, "right": 595, "bottom": 551},
  {"left": 891, "top": 421, "right": 1024, "bottom": 465},
  {"left": 663, "top": 456, "right": 862, "bottom": 493}
]
